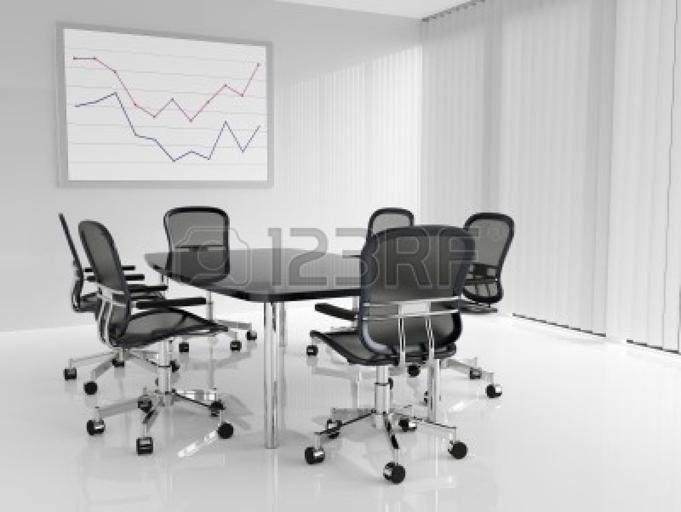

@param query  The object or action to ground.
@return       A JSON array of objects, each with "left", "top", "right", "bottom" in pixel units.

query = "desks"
[{"left": 142, "top": 249, "right": 498, "bottom": 451}]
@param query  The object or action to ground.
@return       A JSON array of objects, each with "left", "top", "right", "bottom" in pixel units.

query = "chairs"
[
  {"left": 55, "top": 212, "right": 190, "bottom": 395},
  {"left": 407, "top": 211, "right": 515, "bottom": 396},
  {"left": 309, "top": 223, "right": 474, "bottom": 480},
  {"left": 162, "top": 205, "right": 258, "bottom": 354},
  {"left": 75, "top": 219, "right": 233, "bottom": 452},
  {"left": 308, "top": 208, "right": 415, "bottom": 361}
]
